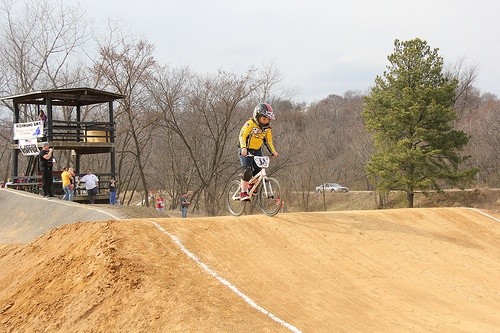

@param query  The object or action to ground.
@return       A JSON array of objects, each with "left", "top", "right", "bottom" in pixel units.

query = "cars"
[{"left": 316, "top": 183, "right": 349, "bottom": 193}]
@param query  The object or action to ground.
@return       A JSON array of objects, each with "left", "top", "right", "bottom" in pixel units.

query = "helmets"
[{"left": 253, "top": 103, "right": 273, "bottom": 130}]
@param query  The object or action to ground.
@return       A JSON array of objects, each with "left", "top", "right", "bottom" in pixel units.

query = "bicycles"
[{"left": 225, "top": 151, "right": 282, "bottom": 217}]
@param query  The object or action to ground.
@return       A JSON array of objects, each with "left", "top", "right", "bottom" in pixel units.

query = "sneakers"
[
  {"left": 240, "top": 191, "right": 251, "bottom": 202},
  {"left": 247, "top": 183, "right": 257, "bottom": 195}
]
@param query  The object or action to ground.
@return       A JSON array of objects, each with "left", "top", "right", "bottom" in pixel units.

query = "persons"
[
  {"left": 37, "top": 110, "right": 47, "bottom": 142},
  {"left": 0, "top": 181, "right": 5, "bottom": 188},
  {"left": 109, "top": 176, "right": 118, "bottom": 205},
  {"left": 181, "top": 193, "right": 190, "bottom": 218},
  {"left": 5, "top": 178, "right": 12, "bottom": 188},
  {"left": 39, "top": 142, "right": 54, "bottom": 197},
  {"left": 62, "top": 167, "right": 75, "bottom": 201},
  {"left": 156, "top": 193, "right": 164, "bottom": 211},
  {"left": 78, "top": 169, "right": 99, "bottom": 204},
  {"left": 238, "top": 103, "right": 279, "bottom": 201}
]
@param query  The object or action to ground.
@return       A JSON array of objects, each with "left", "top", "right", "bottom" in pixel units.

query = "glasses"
[{"left": 266, "top": 112, "right": 273, "bottom": 117}]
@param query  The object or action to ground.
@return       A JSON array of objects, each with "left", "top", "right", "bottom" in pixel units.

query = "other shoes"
[
  {"left": 45, "top": 195, "right": 49, "bottom": 197},
  {"left": 70, "top": 185, "right": 73, "bottom": 190},
  {"left": 48, "top": 194, "right": 56, "bottom": 198}
]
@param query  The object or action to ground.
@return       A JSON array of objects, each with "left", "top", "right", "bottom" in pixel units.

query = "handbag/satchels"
[{"left": 92, "top": 186, "right": 98, "bottom": 195}]
